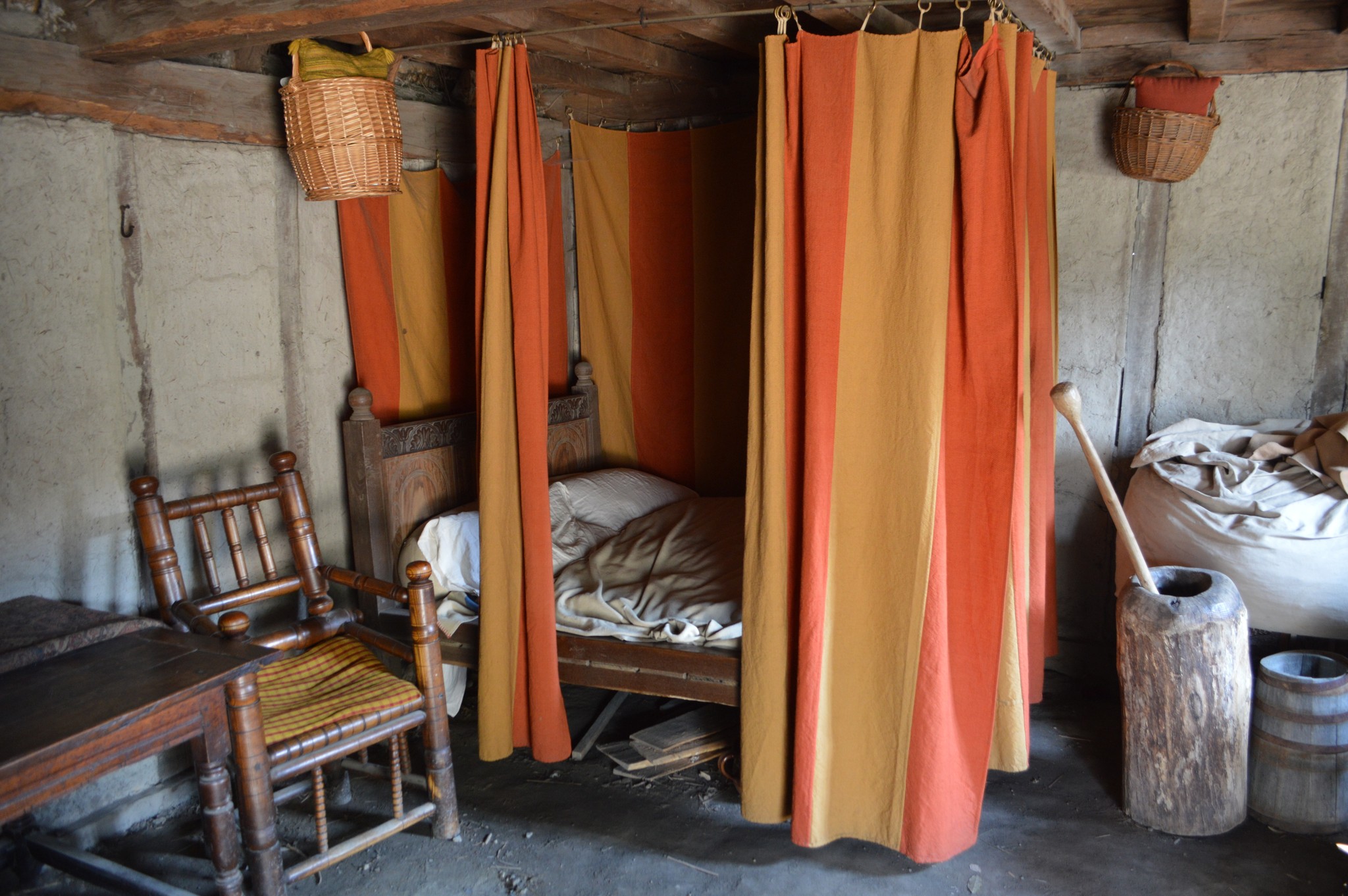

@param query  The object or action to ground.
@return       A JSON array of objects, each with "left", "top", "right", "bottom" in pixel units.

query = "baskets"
[
  {"left": 278, "top": 31, "right": 404, "bottom": 203},
  {"left": 1112, "top": 61, "right": 1221, "bottom": 183}
]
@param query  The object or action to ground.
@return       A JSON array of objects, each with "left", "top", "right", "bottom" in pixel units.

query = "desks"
[{"left": 2, "top": 594, "right": 292, "bottom": 896}]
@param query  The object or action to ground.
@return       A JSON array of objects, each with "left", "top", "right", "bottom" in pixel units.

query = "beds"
[{"left": 339, "top": 348, "right": 741, "bottom": 783}]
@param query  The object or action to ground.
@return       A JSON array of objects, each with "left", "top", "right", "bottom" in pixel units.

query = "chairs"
[{"left": 133, "top": 450, "right": 464, "bottom": 894}]
